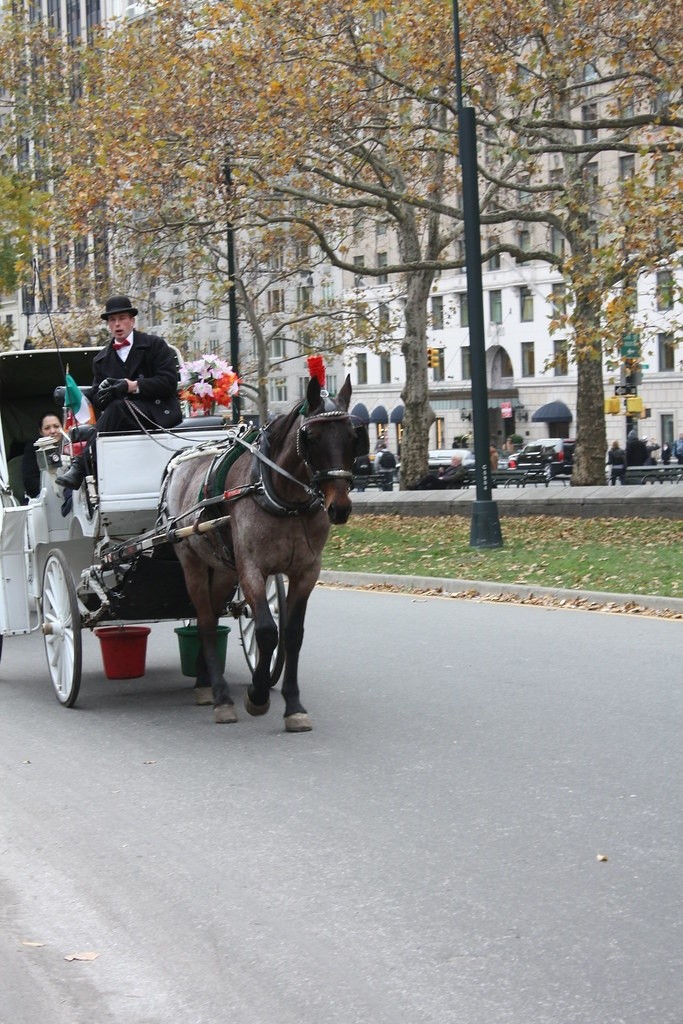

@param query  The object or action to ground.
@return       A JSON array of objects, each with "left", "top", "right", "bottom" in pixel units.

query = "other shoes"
[{"left": 405, "top": 485, "right": 417, "bottom": 490}]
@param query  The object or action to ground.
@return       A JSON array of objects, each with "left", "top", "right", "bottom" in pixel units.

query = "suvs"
[
  {"left": 518, "top": 438, "right": 576, "bottom": 483},
  {"left": 396, "top": 450, "right": 476, "bottom": 479}
]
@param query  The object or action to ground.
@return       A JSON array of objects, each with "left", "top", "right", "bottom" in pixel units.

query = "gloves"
[{"left": 98, "top": 377, "right": 128, "bottom": 404}]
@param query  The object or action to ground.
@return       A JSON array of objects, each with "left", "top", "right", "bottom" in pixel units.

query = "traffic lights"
[{"left": 428, "top": 349, "right": 440, "bottom": 367}]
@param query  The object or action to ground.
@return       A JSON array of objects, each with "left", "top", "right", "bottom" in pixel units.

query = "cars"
[{"left": 507, "top": 453, "right": 520, "bottom": 470}]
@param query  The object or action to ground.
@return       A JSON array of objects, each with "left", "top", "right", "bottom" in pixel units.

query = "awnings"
[
  {"left": 350, "top": 403, "right": 404, "bottom": 425},
  {"left": 531, "top": 401, "right": 573, "bottom": 423}
]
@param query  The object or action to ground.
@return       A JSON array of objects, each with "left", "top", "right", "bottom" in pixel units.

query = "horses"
[{"left": 160, "top": 373, "right": 359, "bottom": 732}]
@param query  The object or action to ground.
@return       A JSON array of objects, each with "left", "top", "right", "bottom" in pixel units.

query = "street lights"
[{"left": 221, "top": 139, "right": 241, "bottom": 425}]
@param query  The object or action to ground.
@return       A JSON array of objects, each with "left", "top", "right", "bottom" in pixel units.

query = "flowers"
[{"left": 177, "top": 353, "right": 243, "bottom": 416}]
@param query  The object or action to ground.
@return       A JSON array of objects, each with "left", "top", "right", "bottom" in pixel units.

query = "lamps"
[
  {"left": 459, "top": 406, "right": 471, "bottom": 422},
  {"left": 515, "top": 401, "right": 528, "bottom": 423}
]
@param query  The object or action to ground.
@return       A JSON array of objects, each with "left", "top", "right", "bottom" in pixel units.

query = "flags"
[{"left": 62, "top": 373, "right": 96, "bottom": 456}]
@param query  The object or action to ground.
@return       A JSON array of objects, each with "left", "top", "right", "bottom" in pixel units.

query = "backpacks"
[{"left": 378, "top": 450, "right": 396, "bottom": 469}]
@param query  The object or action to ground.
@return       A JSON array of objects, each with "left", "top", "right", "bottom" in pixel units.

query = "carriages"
[{"left": 0, "top": 341, "right": 368, "bottom": 733}]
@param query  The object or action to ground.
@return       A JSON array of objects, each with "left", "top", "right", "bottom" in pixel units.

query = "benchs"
[
  {"left": 0, "top": 454, "right": 40, "bottom": 508},
  {"left": 63, "top": 414, "right": 226, "bottom": 459},
  {"left": 351, "top": 466, "right": 683, "bottom": 491}
]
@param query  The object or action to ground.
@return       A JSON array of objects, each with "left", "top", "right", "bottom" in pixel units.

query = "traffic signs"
[
  {"left": 615, "top": 386, "right": 636, "bottom": 396},
  {"left": 621, "top": 332, "right": 641, "bottom": 358}
]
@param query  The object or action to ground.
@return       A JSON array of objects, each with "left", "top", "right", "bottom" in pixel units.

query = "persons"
[
  {"left": 374, "top": 444, "right": 396, "bottom": 491},
  {"left": 54, "top": 297, "right": 185, "bottom": 489},
  {"left": 406, "top": 438, "right": 514, "bottom": 491},
  {"left": 352, "top": 456, "right": 372, "bottom": 494},
  {"left": 22, "top": 412, "right": 64, "bottom": 507},
  {"left": 608, "top": 430, "right": 683, "bottom": 486}
]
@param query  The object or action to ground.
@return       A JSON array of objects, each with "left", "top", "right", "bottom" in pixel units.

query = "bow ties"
[{"left": 112, "top": 340, "right": 130, "bottom": 350}]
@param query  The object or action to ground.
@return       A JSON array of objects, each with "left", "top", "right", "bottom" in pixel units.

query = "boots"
[{"left": 54, "top": 456, "right": 88, "bottom": 490}]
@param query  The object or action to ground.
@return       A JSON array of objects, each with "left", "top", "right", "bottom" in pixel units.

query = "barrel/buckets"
[
  {"left": 173, "top": 619, "right": 231, "bottom": 676},
  {"left": 95, "top": 625, "right": 150, "bottom": 679}
]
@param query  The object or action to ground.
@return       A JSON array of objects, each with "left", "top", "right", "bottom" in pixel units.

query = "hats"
[
  {"left": 379, "top": 444, "right": 386, "bottom": 449},
  {"left": 101, "top": 297, "right": 139, "bottom": 319}
]
